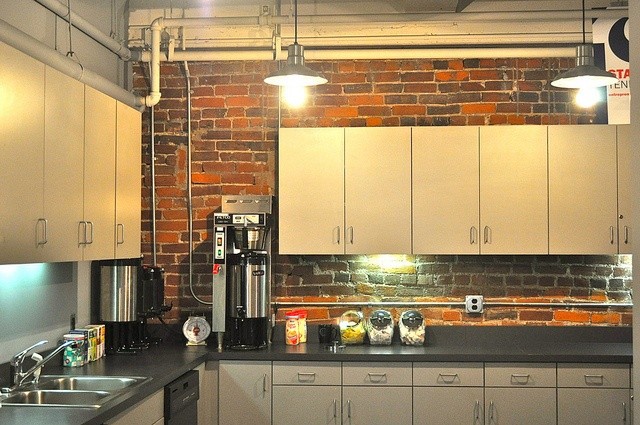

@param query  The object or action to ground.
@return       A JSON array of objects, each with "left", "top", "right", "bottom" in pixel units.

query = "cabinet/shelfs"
[
  {"left": 413, "top": 362, "right": 556, "bottom": 425},
  {"left": 103, "top": 387, "right": 164, "bottom": 424},
  {"left": 205, "top": 360, "right": 272, "bottom": 425},
  {"left": 556, "top": 362, "right": 634, "bottom": 425},
  {"left": 412, "top": 124, "right": 547, "bottom": 254},
  {"left": 272, "top": 361, "right": 413, "bottom": 425},
  {"left": 45, "top": 63, "right": 116, "bottom": 263},
  {"left": 547, "top": 124, "right": 632, "bottom": 255},
  {"left": 0, "top": 41, "right": 46, "bottom": 263},
  {"left": 115, "top": 99, "right": 142, "bottom": 259},
  {"left": 278, "top": 127, "right": 411, "bottom": 255}
]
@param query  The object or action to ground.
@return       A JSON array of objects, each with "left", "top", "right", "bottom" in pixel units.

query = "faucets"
[{"left": 13, "top": 340, "right": 80, "bottom": 388}]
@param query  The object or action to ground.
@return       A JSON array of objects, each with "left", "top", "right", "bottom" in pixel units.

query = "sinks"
[
  {"left": 33, "top": 377, "right": 145, "bottom": 391},
  {"left": 2, "top": 392, "right": 116, "bottom": 406}
]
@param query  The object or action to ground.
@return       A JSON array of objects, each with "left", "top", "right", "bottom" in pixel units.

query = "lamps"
[
  {"left": 263, "top": 1, "right": 329, "bottom": 110},
  {"left": 550, "top": 1, "right": 618, "bottom": 109}
]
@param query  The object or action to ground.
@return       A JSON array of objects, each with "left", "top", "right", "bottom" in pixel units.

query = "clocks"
[{"left": 182, "top": 317, "right": 212, "bottom": 348}]
